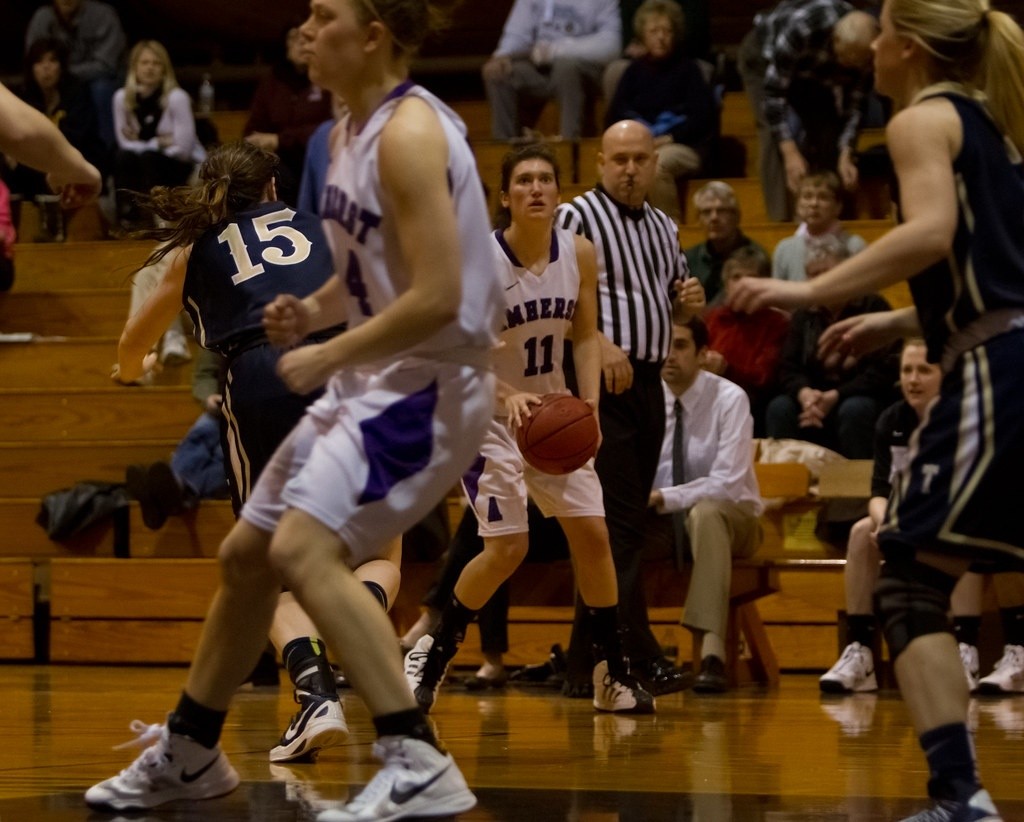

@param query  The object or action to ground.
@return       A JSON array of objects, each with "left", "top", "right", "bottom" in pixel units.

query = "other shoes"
[
  {"left": 125, "top": 459, "right": 180, "bottom": 531},
  {"left": 161, "top": 331, "right": 192, "bottom": 366},
  {"left": 465, "top": 674, "right": 508, "bottom": 689}
]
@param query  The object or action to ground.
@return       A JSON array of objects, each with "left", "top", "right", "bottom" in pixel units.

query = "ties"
[{"left": 672, "top": 400, "right": 686, "bottom": 550}]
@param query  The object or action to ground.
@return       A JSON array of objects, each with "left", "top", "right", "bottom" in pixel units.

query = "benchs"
[{"left": 1, "top": 92, "right": 913, "bottom": 671}]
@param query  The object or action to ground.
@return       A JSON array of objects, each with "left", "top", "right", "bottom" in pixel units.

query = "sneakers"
[
  {"left": 315, "top": 736, "right": 478, "bottom": 821},
  {"left": 819, "top": 641, "right": 878, "bottom": 693},
  {"left": 958, "top": 642, "right": 978, "bottom": 692},
  {"left": 898, "top": 774, "right": 1002, "bottom": 822},
  {"left": 269, "top": 690, "right": 348, "bottom": 762},
  {"left": 977, "top": 645, "right": 1024, "bottom": 693},
  {"left": 84, "top": 720, "right": 239, "bottom": 814},
  {"left": 404, "top": 634, "right": 459, "bottom": 713},
  {"left": 593, "top": 656, "right": 657, "bottom": 713}
]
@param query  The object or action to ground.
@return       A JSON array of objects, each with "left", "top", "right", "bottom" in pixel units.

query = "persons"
[
  {"left": 770, "top": 170, "right": 869, "bottom": 325},
  {"left": 755, "top": 241, "right": 906, "bottom": 460},
  {"left": 0, "top": 80, "right": 103, "bottom": 301},
  {"left": 0, "top": 0, "right": 336, "bottom": 243},
  {"left": 680, "top": 181, "right": 791, "bottom": 393},
  {"left": 484, "top": 0, "right": 624, "bottom": 147},
  {"left": 396, "top": 495, "right": 507, "bottom": 690},
  {"left": 235, "top": 639, "right": 283, "bottom": 694},
  {"left": 978, "top": 571, "right": 1024, "bottom": 696},
  {"left": 548, "top": 120, "right": 707, "bottom": 698},
  {"left": 628, "top": 314, "right": 764, "bottom": 695},
  {"left": 126, "top": 350, "right": 230, "bottom": 532},
  {"left": 111, "top": 138, "right": 401, "bottom": 764},
  {"left": 115, "top": 237, "right": 193, "bottom": 387},
  {"left": 85, "top": 2, "right": 503, "bottom": 822},
  {"left": 408, "top": 146, "right": 658, "bottom": 715},
  {"left": 735, "top": 0, "right": 879, "bottom": 223},
  {"left": 724, "top": 0, "right": 1024, "bottom": 822},
  {"left": 608, "top": 0, "right": 716, "bottom": 222},
  {"left": 819, "top": 337, "right": 980, "bottom": 695}
]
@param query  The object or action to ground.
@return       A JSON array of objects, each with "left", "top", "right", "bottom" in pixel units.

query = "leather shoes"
[
  {"left": 636, "top": 661, "right": 691, "bottom": 698},
  {"left": 693, "top": 653, "right": 727, "bottom": 691}
]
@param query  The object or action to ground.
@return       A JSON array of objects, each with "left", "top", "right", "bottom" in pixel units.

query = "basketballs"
[{"left": 517, "top": 393, "right": 600, "bottom": 475}]
